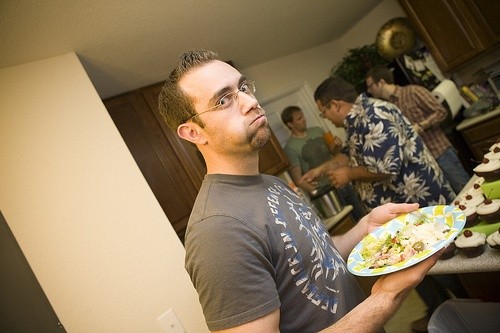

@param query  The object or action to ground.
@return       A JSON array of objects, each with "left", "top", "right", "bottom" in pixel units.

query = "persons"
[
  {"left": 279, "top": 65, "right": 469, "bottom": 214},
  {"left": 158, "top": 49, "right": 444, "bottom": 333}
]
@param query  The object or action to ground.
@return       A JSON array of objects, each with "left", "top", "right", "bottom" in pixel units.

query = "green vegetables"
[{"left": 361, "top": 213, "right": 428, "bottom": 268}]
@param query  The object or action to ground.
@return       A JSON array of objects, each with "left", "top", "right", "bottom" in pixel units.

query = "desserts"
[
  {"left": 473, "top": 142, "right": 500, "bottom": 182},
  {"left": 439, "top": 227, "right": 500, "bottom": 260},
  {"left": 450, "top": 177, "right": 500, "bottom": 228}
]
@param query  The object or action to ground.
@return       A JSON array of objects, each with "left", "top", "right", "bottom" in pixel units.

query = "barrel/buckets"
[{"left": 310, "top": 185, "right": 343, "bottom": 218}]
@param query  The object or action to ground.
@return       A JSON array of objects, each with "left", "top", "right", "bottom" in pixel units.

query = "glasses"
[
  {"left": 185, "top": 81, "right": 257, "bottom": 124},
  {"left": 367, "top": 77, "right": 383, "bottom": 93},
  {"left": 319, "top": 97, "right": 340, "bottom": 119}
]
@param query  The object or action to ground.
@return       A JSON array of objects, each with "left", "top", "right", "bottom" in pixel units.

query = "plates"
[{"left": 347, "top": 204, "right": 466, "bottom": 276}]
